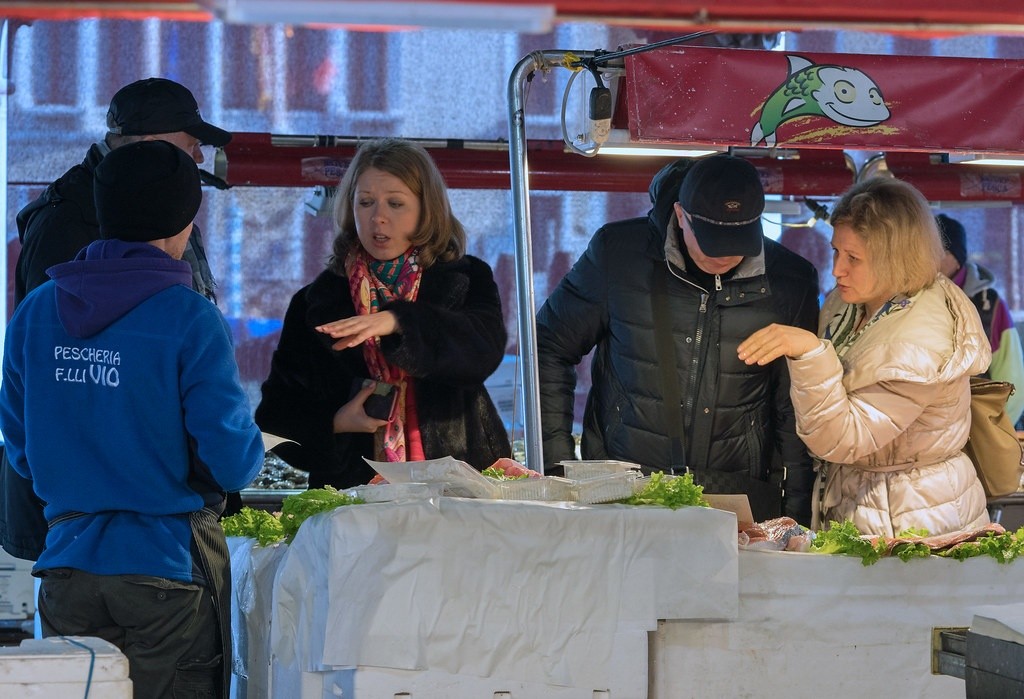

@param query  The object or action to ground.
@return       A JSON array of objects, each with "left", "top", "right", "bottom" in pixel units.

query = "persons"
[
  {"left": 537, "top": 152, "right": 1024, "bottom": 542},
  {"left": 255, "top": 139, "right": 517, "bottom": 490},
  {"left": 0, "top": 139, "right": 264, "bottom": 698},
  {"left": 0, "top": 78, "right": 234, "bottom": 638}
]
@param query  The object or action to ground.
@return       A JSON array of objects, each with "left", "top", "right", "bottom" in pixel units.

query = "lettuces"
[
  {"left": 616, "top": 470, "right": 711, "bottom": 511},
  {"left": 219, "top": 483, "right": 365, "bottom": 548},
  {"left": 799, "top": 518, "right": 1024, "bottom": 567},
  {"left": 481, "top": 466, "right": 529, "bottom": 481}
]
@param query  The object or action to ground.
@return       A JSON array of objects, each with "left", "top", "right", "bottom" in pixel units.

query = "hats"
[
  {"left": 679, "top": 154, "right": 766, "bottom": 258},
  {"left": 106, "top": 77, "right": 232, "bottom": 147},
  {"left": 935, "top": 213, "right": 967, "bottom": 267},
  {"left": 93, "top": 140, "right": 202, "bottom": 242}
]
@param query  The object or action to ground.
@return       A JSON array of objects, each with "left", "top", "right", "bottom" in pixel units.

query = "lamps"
[{"left": 303, "top": 184, "right": 326, "bottom": 218}]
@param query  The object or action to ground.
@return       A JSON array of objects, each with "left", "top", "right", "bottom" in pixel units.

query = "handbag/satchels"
[{"left": 961, "top": 377, "right": 1024, "bottom": 498}]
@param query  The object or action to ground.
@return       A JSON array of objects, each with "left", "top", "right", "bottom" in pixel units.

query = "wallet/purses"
[{"left": 362, "top": 378, "right": 402, "bottom": 423}]
